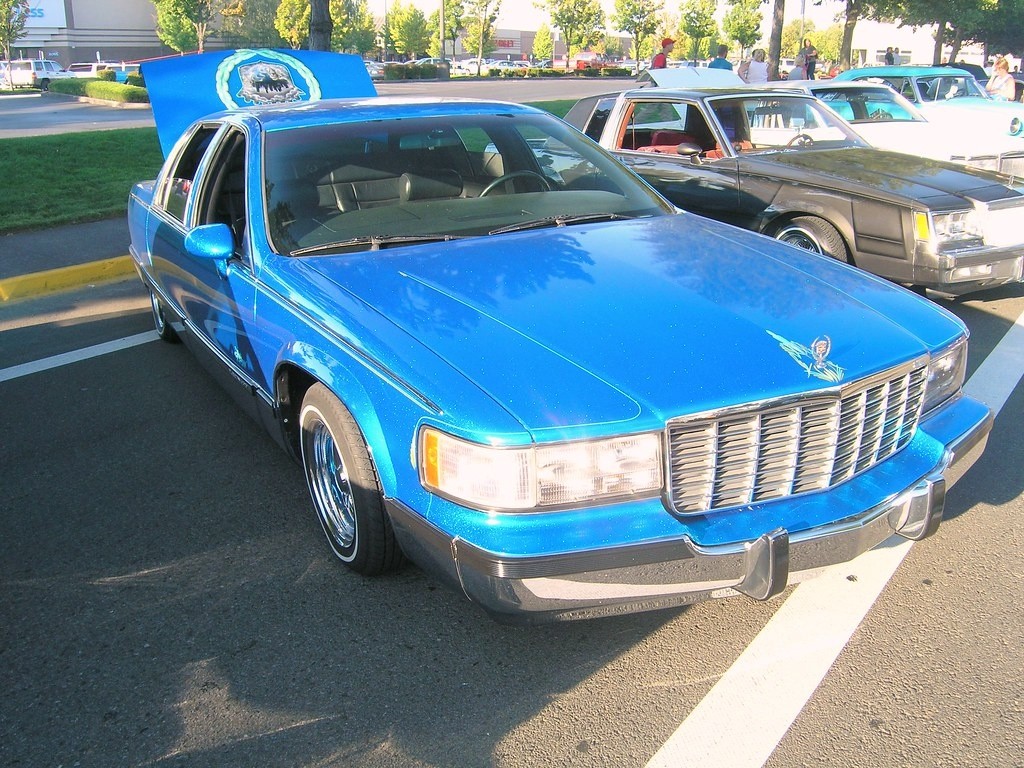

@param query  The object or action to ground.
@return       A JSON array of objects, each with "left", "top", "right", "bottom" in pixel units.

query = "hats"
[{"left": 662, "top": 38, "right": 677, "bottom": 47}]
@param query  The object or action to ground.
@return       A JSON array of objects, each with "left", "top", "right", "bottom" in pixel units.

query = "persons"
[
  {"left": 1014, "top": 65, "right": 1019, "bottom": 73},
  {"left": 709, "top": 45, "right": 732, "bottom": 71},
  {"left": 984, "top": 60, "right": 994, "bottom": 76},
  {"left": 943, "top": 57, "right": 947, "bottom": 63},
  {"left": 885, "top": 48, "right": 901, "bottom": 66},
  {"left": 738, "top": 49, "right": 772, "bottom": 83},
  {"left": 651, "top": 38, "right": 676, "bottom": 69},
  {"left": 787, "top": 58, "right": 806, "bottom": 80},
  {"left": 799, "top": 38, "right": 817, "bottom": 80},
  {"left": 985, "top": 58, "right": 1015, "bottom": 101}
]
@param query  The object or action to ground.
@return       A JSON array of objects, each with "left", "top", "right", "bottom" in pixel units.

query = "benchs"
[{"left": 184, "top": 137, "right": 391, "bottom": 225}]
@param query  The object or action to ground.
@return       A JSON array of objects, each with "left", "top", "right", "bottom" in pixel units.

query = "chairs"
[
  {"left": 397, "top": 167, "right": 465, "bottom": 205},
  {"left": 275, "top": 179, "right": 331, "bottom": 244},
  {"left": 624, "top": 132, "right": 754, "bottom": 159}
]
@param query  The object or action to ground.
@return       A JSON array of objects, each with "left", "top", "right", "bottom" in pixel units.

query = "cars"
[
  {"left": 126, "top": 46, "right": 997, "bottom": 628},
  {"left": 631, "top": 62, "right": 1024, "bottom": 178},
  {"left": 1, "top": 53, "right": 560, "bottom": 86},
  {"left": 563, "top": 89, "right": 1023, "bottom": 297}
]
